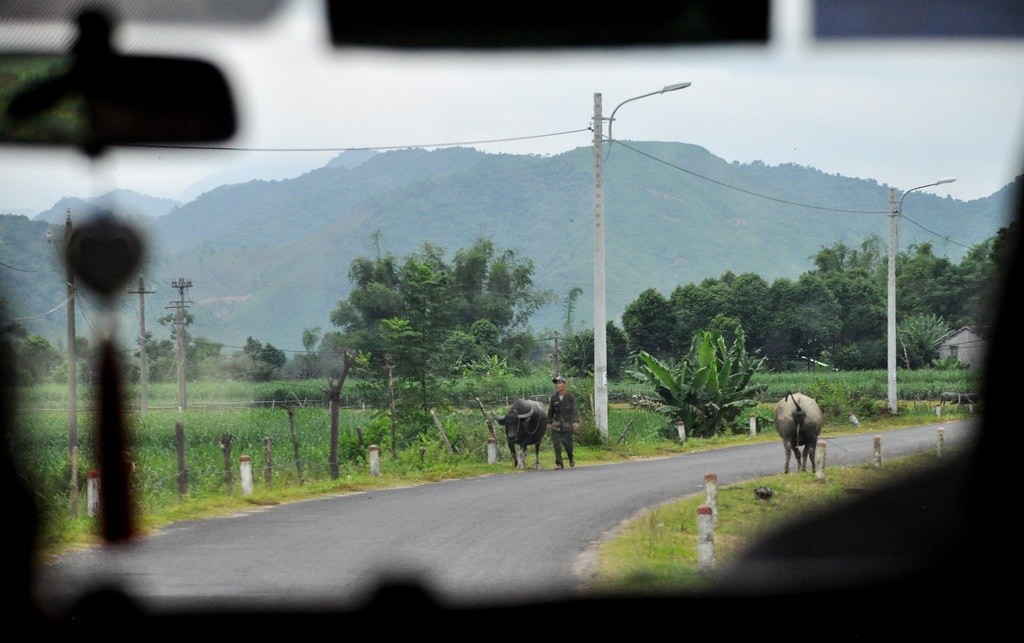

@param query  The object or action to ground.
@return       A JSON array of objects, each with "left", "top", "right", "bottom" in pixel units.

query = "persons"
[{"left": 546, "top": 376, "right": 581, "bottom": 470}]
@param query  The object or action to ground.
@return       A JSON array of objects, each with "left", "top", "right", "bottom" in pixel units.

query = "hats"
[{"left": 552, "top": 377, "right": 566, "bottom": 385}]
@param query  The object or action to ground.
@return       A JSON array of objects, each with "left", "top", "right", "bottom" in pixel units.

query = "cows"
[
  {"left": 939, "top": 392, "right": 979, "bottom": 405},
  {"left": 491, "top": 399, "right": 547, "bottom": 470},
  {"left": 775, "top": 390, "right": 824, "bottom": 474}
]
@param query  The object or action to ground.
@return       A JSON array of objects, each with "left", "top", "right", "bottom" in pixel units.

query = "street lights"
[
  {"left": 593, "top": 82, "right": 692, "bottom": 440},
  {"left": 885, "top": 180, "right": 956, "bottom": 413}
]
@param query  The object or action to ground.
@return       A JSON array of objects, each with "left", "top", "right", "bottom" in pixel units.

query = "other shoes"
[
  {"left": 555, "top": 464, "right": 563, "bottom": 469},
  {"left": 569, "top": 458, "right": 575, "bottom": 467}
]
